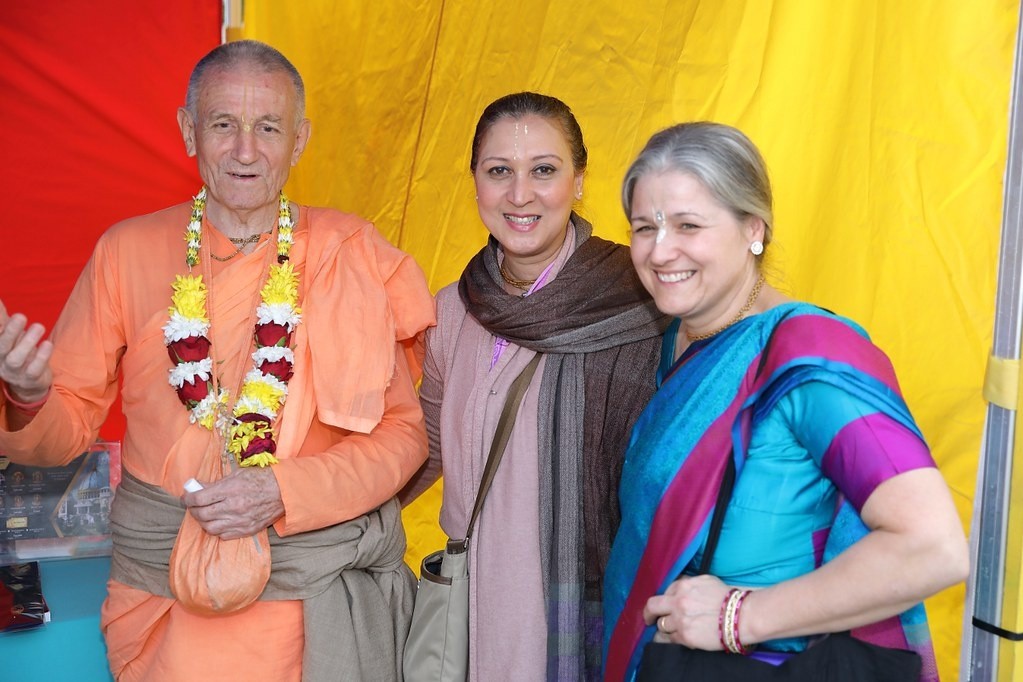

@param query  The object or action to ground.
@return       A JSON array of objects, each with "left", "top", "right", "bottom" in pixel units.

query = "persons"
[
  {"left": 599, "top": 121, "right": 970, "bottom": 682},
  {"left": 394, "top": 92, "right": 671, "bottom": 682},
  {"left": 0, "top": 41, "right": 437, "bottom": 682}
]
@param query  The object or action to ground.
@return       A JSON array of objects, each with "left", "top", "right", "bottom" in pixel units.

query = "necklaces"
[
  {"left": 501, "top": 264, "right": 536, "bottom": 291},
  {"left": 686, "top": 274, "right": 765, "bottom": 341},
  {"left": 165, "top": 186, "right": 301, "bottom": 471}
]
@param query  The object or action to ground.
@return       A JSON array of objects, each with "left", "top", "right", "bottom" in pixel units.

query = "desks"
[{"left": 0, "top": 555, "right": 114, "bottom": 682}]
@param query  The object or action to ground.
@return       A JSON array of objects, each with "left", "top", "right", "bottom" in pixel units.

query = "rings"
[{"left": 661, "top": 617, "right": 666, "bottom": 629}]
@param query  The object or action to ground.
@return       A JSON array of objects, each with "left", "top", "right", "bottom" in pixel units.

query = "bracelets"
[
  {"left": 2, "top": 382, "right": 51, "bottom": 415},
  {"left": 718, "top": 588, "right": 757, "bottom": 656}
]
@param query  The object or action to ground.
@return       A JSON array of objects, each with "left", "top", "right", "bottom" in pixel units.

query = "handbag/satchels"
[
  {"left": 631, "top": 304, "right": 924, "bottom": 682},
  {"left": 401, "top": 538, "right": 472, "bottom": 682}
]
